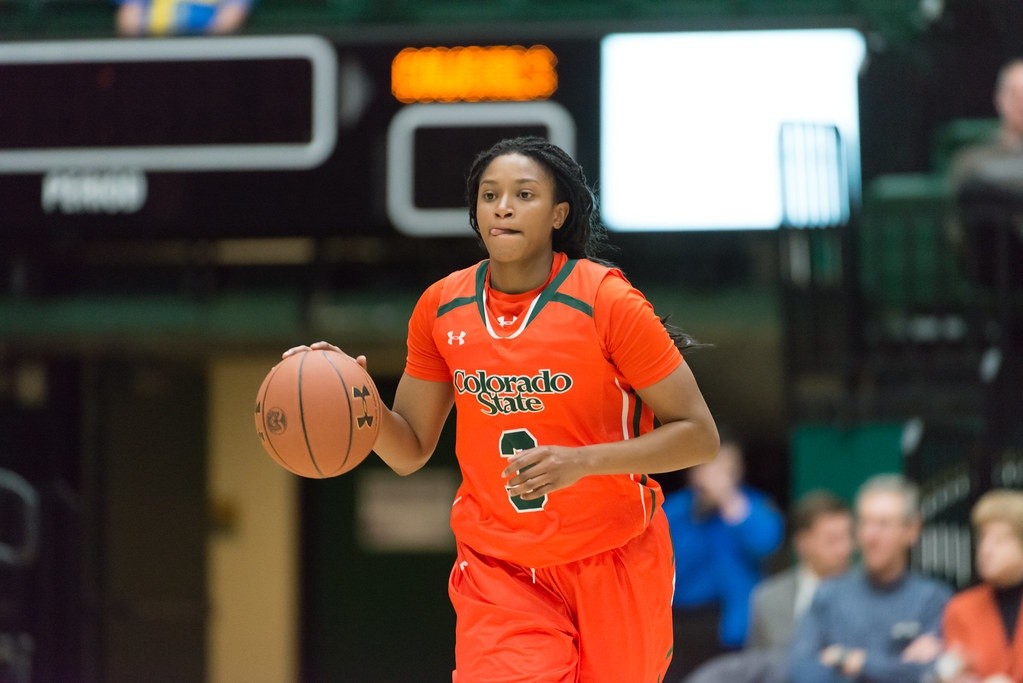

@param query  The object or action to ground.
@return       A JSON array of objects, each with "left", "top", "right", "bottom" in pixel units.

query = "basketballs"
[{"left": 251, "top": 349, "right": 382, "bottom": 480}]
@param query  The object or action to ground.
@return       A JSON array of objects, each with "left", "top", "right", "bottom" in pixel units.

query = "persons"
[
  {"left": 661, "top": 436, "right": 1022, "bottom": 683},
  {"left": 281, "top": 134, "right": 720, "bottom": 683}
]
